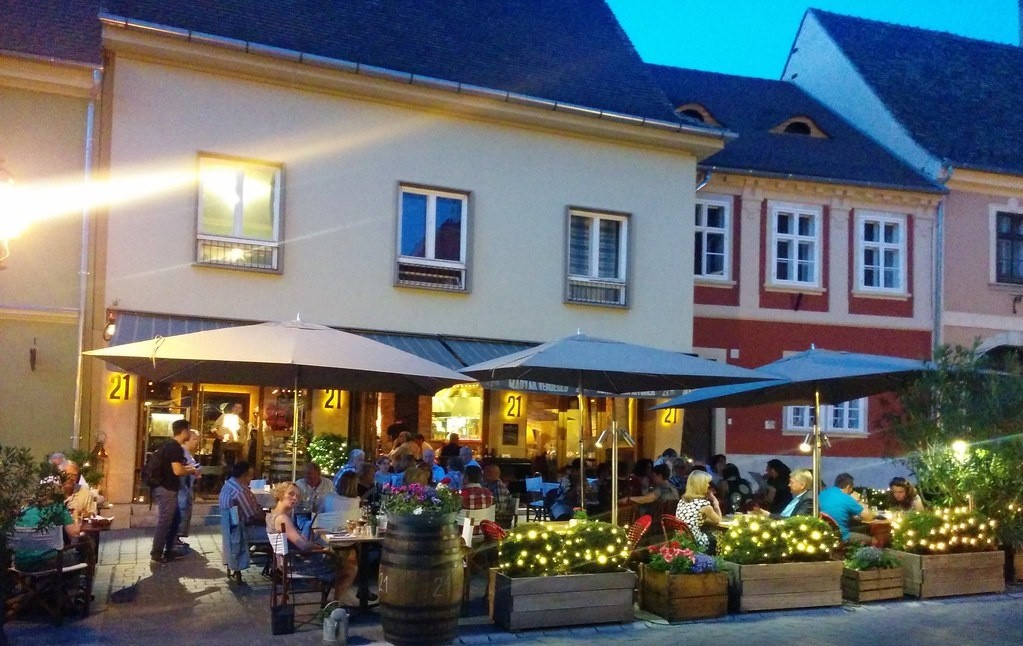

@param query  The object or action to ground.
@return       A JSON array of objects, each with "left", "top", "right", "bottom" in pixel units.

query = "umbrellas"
[
  {"left": 648, "top": 342, "right": 1012, "bottom": 520},
  {"left": 82, "top": 311, "right": 479, "bottom": 476},
  {"left": 457, "top": 325, "right": 791, "bottom": 523}
]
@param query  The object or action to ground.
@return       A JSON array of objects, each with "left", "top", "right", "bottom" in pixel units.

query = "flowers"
[
  {"left": 382, "top": 482, "right": 465, "bottom": 517},
  {"left": 648, "top": 542, "right": 730, "bottom": 574},
  {"left": 845, "top": 546, "right": 904, "bottom": 570}
]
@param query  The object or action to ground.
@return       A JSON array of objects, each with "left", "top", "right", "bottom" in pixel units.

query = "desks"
[
  {"left": 78, "top": 520, "right": 111, "bottom": 548},
  {"left": 318, "top": 533, "right": 385, "bottom": 614}
]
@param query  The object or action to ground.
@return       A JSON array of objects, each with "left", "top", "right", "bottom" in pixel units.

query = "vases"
[
  {"left": 638, "top": 562, "right": 728, "bottom": 621},
  {"left": 377, "top": 512, "right": 464, "bottom": 646},
  {"left": 841, "top": 564, "right": 905, "bottom": 604}
]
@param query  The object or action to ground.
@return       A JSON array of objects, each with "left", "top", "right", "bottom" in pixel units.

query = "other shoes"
[
  {"left": 166, "top": 540, "right": 189, "bottom": 551},
  {"left": 356, "top": 588, "right": 378, "bottom": 601},
  {"left": 151, "top": 552, "right": 173, "bottom": 563},
  {"left": 336, "top": 592, "right": 361, "bottom": 607}
]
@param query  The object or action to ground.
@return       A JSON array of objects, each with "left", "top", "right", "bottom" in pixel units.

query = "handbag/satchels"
[{"left": 271, "top": 604, "right": 295, "bottom": 634}]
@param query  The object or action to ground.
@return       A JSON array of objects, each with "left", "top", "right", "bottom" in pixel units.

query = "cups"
[{"left": 871, "top": 506, "right": 878, "bottom": 512}]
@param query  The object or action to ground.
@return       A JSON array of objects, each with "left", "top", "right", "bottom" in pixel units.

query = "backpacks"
[{"left": 145, "top": 440, "right": 176, "bottom": 488}]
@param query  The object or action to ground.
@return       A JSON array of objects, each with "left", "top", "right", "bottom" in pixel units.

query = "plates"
[{"left": 329, "top": 531, "right": 347, "bottom": 536}]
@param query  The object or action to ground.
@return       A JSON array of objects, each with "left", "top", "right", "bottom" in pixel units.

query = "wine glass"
[{"left": 731, "top": 492, "right": 740, "bottom": 513}]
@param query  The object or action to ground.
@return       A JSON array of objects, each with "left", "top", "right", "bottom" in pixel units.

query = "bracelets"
[
  {"left": 858, "top": 500, "right": 861, "bottom": 502},
  {"left": 713, "top": 501, "right": 718, "bottom": 505}
]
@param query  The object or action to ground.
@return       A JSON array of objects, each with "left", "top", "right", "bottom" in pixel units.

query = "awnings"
[
  {"left": 443, "top": 339, "right": 690, "bottom": 399},
  {"left": 106, "top": 313, "right": 467, "bottom": 373}
]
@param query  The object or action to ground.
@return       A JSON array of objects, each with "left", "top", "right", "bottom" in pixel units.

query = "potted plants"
[
  {"left": 724, "top": 512, "right": 846, "bottom": 613},
  {"left": 485, "top": 516, "right": 637, "bottom": 632},
  {"left": 887, "top": 506, "right": 1007, "bottom": 600}
]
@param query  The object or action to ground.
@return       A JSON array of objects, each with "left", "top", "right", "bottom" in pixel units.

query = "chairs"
[
  {"left": 462, "top": 471, "right": 842, "bottom": 566},
  {"left": 5, "top": 525, "right": 94, "bottom": 622},
  {"left": 220, "top": 499, "right": 274, "bottom": 582},
  {"left": 267, "top": 522, "right": 336, "bottom": 611}
]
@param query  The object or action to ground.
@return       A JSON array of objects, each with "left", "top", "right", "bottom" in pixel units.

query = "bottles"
[
  {"left": 367, "top": 500, "right": 389, "bottom": 539},
  {"left": 860, "top": 493, "right": 869, "bottom": 517}
]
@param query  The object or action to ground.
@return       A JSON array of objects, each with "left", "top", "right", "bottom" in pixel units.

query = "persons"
[
  {"left": 885, "top": 477, "right": 924, "bottom": 512},
  {"left": 818, "top": 473, "right": 877, "bottom": 547},
  {"left": 150, "top": 419, "right": 201, "bottom": 563},
  {"left": 247, "top": 407, "right": 270, "bottom": 463},
  {"left": 266, "top": 415, "right": 512, "bottom": 607},
  {"left": 676, "top": 471, "right": 722, "bottom": 555},
  {"left": 550, "top": 448, "right": 792, "bottom": 521},
  {"left": 219, "top": 461, "right": 268, "bottom": 539},
  {"left": 6, "top": 452, "right": 99, "bottom": 603},
  {"left": 210, "top": 403, "right": 246, "bottom": 466},
  {"left": 754, "top": 468, "right": 814, "bottom": 517}
]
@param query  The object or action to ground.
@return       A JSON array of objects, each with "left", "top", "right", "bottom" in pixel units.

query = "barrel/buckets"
[
  {"left": 270, "top": 454, "right": 305, "bottom": 483},
  {"left": 323, "top": 600, "right": 349, "bottom": 643},
  {"left": 381, "top": 522, "right": 464, "bottom": 645}
]
